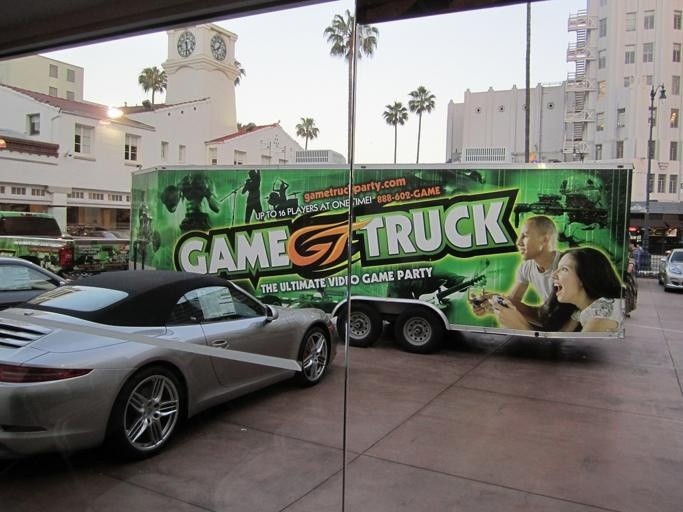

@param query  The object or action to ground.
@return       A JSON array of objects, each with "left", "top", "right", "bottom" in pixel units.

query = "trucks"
[{"left": 1, "top": 211, "right": 130, "bottom": 277}]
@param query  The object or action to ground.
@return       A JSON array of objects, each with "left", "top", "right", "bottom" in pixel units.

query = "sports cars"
[{"left": 1, "top": 269, "right": 338, "bottom": 461}]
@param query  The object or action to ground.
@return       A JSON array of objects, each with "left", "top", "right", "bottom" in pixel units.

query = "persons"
[
  {"left": 468, "top": 216, "right": 565, "bottom": 328},
  {"left": 242, "top": 170, "right": 264, "bottom": 224},
  {"left": 627, "top": 240, "right": 640, "bottom": 276},
  {"left": 488, "top": 247, "right": 626, "bottom": 332},
  {"left": 273, "top": 179, "right": 289, "bottom": 203}
]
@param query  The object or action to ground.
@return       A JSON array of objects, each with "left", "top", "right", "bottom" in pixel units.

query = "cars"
[
  {"left": 657, "top": 248, "right": 683, "bottom": 293},
  {"left": 0, "top": 255, "right": 69, "bottom": 309},
  {"left": 74, "top": 230, "right": 132, "bottom": 239}
]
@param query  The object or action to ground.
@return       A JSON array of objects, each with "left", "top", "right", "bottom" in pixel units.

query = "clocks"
[
  {"left": 208, "top": 32, "right": 227, "bottom": 63},
  {"left": 176, "top": 31, "right": 197, "bottom": 58}
]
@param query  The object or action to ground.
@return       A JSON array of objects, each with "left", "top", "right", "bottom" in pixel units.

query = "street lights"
[{"left": 637, "top": 83, "right": 667, "bottom": 271}]
